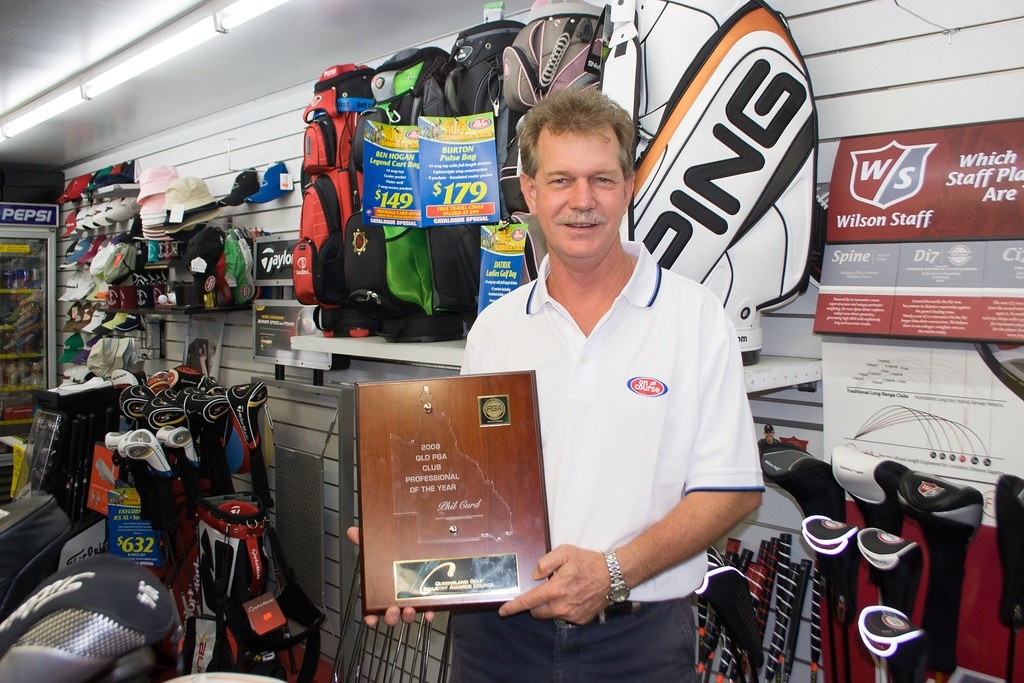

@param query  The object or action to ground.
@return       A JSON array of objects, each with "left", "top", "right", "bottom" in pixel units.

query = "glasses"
[{"left": 766, "top": 430, "right": 773, "bottom": 433}]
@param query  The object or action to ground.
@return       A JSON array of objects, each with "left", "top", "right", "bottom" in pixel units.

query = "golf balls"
[{"left": 158, "top": 294, "right": 167, "bottom": 304}]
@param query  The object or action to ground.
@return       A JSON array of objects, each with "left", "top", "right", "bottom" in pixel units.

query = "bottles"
[{"left": 0, "top": 265, "right": 42, "bottom": 385}]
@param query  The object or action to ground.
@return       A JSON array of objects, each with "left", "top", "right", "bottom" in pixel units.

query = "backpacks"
[
  {"left": 292, "top": 1, "right": 609, "bottom": 343},
  {"left": 161, "top": 482, "right": 322, "bottom": 682}
]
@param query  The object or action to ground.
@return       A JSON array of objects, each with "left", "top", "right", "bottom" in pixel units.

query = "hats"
[
  {"left": 56, "top": 160, "right": 140, "bottom": 387},
  {"left": 0, "top": 290, "right": 48, "bottom": 384},
  {"left": 764, "top": 424, "right": 773, "bottom": 430},
  {"left": 134, "top": 162, "right": 295, "bottom": 313}
]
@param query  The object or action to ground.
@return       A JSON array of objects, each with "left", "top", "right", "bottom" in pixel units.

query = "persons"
[
  {"left": 757, "top": 424, "right": 782, "bottom": 452},
  {"left": 347, "top": 86, "right": 764, "bottom": 682}
]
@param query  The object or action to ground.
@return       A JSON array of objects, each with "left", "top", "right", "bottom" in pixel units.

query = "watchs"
[{"left": 603, "top": 550, "right": 630, "bottom": 604}]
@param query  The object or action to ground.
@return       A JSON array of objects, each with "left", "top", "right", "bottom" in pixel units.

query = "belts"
[{"left": 540, "top": 600, "right": 633, "bottom": 628}]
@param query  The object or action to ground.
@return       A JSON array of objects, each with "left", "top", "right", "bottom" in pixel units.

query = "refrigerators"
[{"left": 0, "top": 202, "right": 58, "bottom": 505}]
